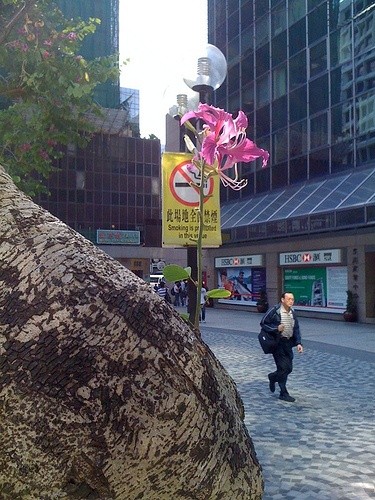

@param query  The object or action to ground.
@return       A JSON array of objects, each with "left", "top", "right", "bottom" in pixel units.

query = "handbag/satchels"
[{"left": 258, "top": 327, "right": 278, "bottom": 354}]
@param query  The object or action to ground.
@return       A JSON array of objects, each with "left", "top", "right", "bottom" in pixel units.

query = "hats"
[{"left": 158, "top": 282, "right": 165, "bottom": 285}]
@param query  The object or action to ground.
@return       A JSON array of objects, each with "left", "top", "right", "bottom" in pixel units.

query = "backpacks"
[
  {"left": 165, "top": 288, "right": 172, "bottom": 304},
  {"left": 171, "top": 287, "right": 175, "bottom": 296}
]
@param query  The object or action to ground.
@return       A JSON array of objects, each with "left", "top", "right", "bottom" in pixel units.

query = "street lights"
[{"left": 172, "top": 41, "right": 227, "bottom": 329}]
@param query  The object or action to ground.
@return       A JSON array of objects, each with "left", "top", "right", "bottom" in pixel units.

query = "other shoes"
[
  {"left": 202, "top": 320, "right": 206, "bottom": 323},
  {"left": 268, "top": 373, "right": 275, "bottom": 393},
  {"left": 279, "top": 392, "right": 296, "bottom": 402},
  {"left": 178, "top": 305, "right": 182, "bottom": 307},
  {"left": 174, "top": 306, "right": 177, "bottom": 307},
  {"left": 199, "top": 321, "right": 202, "bottom": 323}
]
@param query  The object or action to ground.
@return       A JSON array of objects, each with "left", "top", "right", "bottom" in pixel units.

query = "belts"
[{"left": 281, "top": 337, "right": 293, "bottom": 340}]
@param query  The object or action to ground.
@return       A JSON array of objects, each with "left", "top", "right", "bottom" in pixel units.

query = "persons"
[
  {"left": 154, "top": 278, "right": 208, "bottom": 324},
  {"left": 260, "top": 291, "right": 303, "bottom": 402},
  {"left": 220, "top": 269, "right": 246, "bottom": 300}
]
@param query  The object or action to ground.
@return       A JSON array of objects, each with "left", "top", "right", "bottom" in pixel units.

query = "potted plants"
[
  {"left": 343, "top": 290, "right": 358, "bottom": 321},
  {"left": 256, "top": 289, "right": 268, "bottom": 312}
]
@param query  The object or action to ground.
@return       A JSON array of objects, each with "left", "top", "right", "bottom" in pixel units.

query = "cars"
[{"left": 149, "top": 275, "right": 164, "bottom": 289}]
